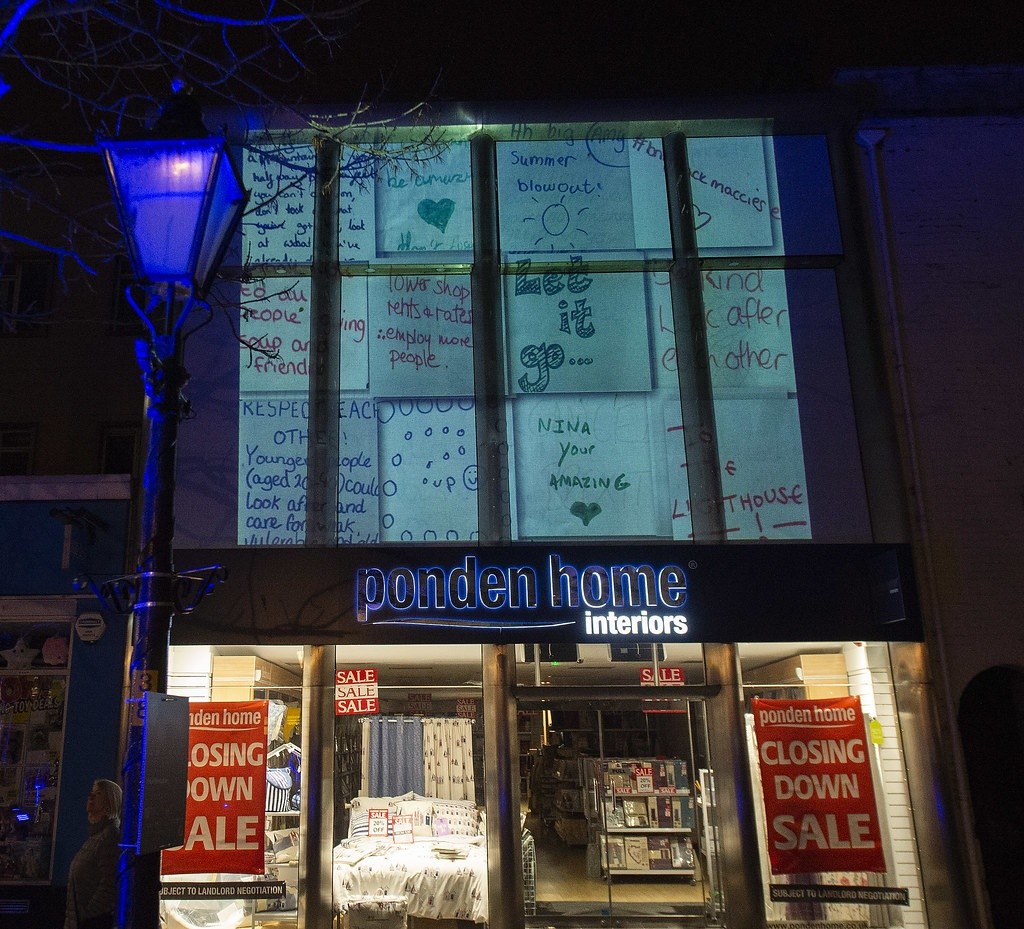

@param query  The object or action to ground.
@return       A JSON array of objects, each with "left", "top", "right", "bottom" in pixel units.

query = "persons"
[{"left": 64, "top": 780, "right": 121, "bottom": 928}]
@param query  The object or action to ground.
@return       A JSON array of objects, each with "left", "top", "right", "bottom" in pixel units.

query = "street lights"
[{"left": 97, "top": 135, "right": 256, "bottom": 929}]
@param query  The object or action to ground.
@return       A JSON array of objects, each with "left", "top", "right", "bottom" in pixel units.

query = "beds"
[
  {"left": 254, "top": 826, "right": 299, "bottom": 913},
  {"left": 331, "top": 790, "right": 489, "bottom": 929}
]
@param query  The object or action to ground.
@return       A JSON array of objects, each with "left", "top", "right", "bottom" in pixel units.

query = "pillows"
[
  {"left": 265, "top": 831, "right": 298, "bottom": 864},
  {"left": 349, "top": 791, "right": 481, "bottom": 838}
]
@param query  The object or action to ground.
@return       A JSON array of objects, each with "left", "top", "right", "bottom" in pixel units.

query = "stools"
[{"left": 343, "top": 895, "right": 408, "bottom": 929}]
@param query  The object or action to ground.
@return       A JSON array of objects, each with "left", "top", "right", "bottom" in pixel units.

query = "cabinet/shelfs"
[
  {"left": 211, "top": 655, "right": 302, "bottom": 701},
  {"left": 517, "top": 729, "right": 701, "bottom": 884},
  {"left": 742, "top": 653, "right": 849, "bottom": 701}
]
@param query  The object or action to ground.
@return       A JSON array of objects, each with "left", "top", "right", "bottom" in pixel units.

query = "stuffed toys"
[{"left": 0, "top": 634, "right": 68, "bottom": 669}]
